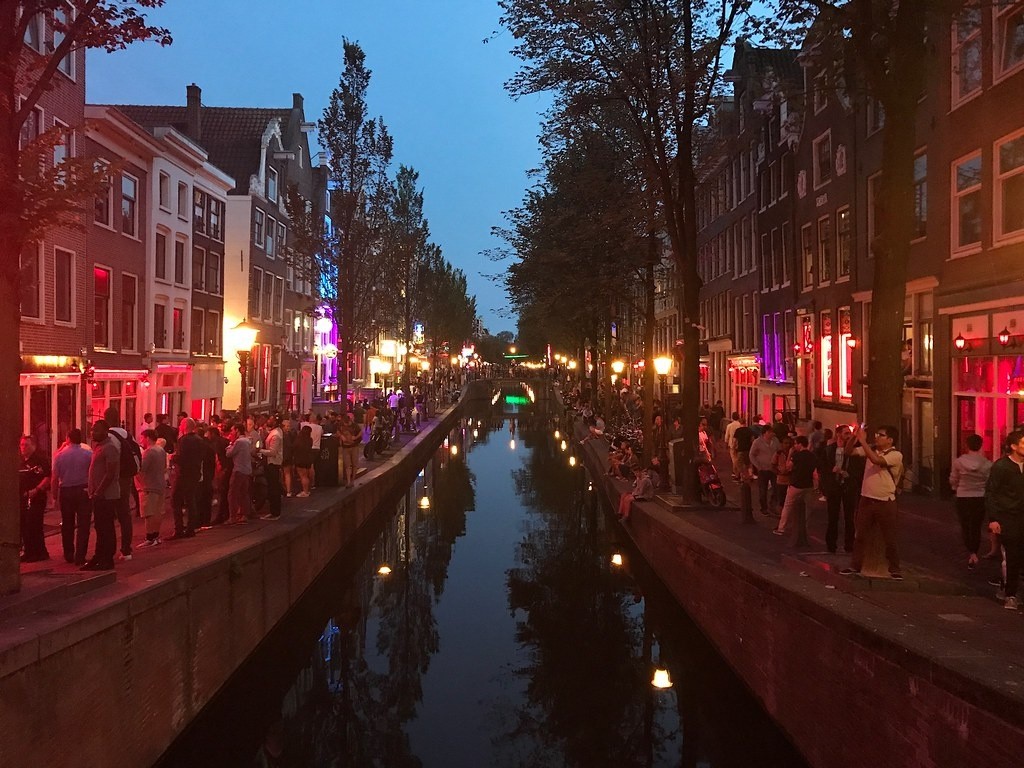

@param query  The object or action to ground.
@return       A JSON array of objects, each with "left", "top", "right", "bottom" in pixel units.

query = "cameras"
[{"left": 860, "top": 423, "right": 867, "bottom": 431}]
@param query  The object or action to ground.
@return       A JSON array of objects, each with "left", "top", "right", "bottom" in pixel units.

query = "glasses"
[{"left": 875, "top": 431, "right": 888, "bottom": 437}]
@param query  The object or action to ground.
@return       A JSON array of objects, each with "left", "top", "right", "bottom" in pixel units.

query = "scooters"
[{"left": 688, "top": 451, "right": 727, "bottom": 510}]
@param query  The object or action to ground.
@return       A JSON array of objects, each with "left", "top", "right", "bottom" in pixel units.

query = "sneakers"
[
  {"left": 838, "top": 565, "right": 858, "bottom": 575},
  {"left": 286, "top": 480, "right": 356, "bottom": 497},
  {"left": 260, "top": 513, "right": 280, "bottom": 520},
  {"left": 986, "top": 574, "right": 1003, "bottom": 588},
  {"left": 137, "top": 538, "right": 160, "bottom": 548},
  {"left": 996, "top": 588, "right": 1019, "bottom": 609},
  {"left": 891, "top": 572, "right": 903, "bottom": 580},
  {"left": 967, "top": 552, "right": 979, "bottom": 571}
]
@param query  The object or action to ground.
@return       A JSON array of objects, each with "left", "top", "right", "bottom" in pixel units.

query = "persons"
[
  {"left": 447, "top": 364, "right": 549, "bottom": 390},
  {"left": 550, "top": 367, "right": 723, "bottom": 525},
  {"left": 19, "top": 367, "right": 446, "bottom": 571},
  {"left": 724, "top": 412, "right": 1024, "bottom": 607}
]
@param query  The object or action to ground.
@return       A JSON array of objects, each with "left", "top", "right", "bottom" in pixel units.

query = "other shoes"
[
  {"left": 773, "top": 528, "right": 784, "bottom": 534},
  {"left": 18, "top": 550, "right": 133, "bottom": 570},
  {"left": 761, "top": 511, "right": 770, "bottom": 516},
  {"left": 602, "top": 464, "right": 630, "bottom": 526},
  {"left": 844, "top": 544, "right": 854, "bottom": 553},
  {"left": 750, "top": 474, "right": 758, "bottom": 479},
  {"left": 819, "top": 496, "right": 826, "bottom": 501},
  {"left": 826, "top": 543, "right": 836, "bottom": 551},
  {"left": 731, "top": 478, "right": 739, "bottom": 483}
]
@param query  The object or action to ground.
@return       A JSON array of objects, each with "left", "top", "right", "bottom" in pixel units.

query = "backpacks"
[{"left": 109, "top": 429, "right": 142, "bottom": 478}]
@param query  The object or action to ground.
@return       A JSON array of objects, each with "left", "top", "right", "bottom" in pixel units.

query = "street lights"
[
  {"left": 652, "top": 351, "right": 674, "bottom": 491},
  {"left": 381, "top": 361, "right": 392, "bottom": 397},
  {"left": 230, "top": 317, "right": 261, "bottom": 420},
  {"left": 421, "top": 361, "right": 430, "bottom": 421},
  {"left": 611, "top": 359, "right": 625, "bottom": 422}
]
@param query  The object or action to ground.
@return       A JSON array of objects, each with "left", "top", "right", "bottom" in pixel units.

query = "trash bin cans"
[
  {"left": 312, "top": 434, "right": 341, "bottom": 486},
  {"left": 668, "top": 437, "right": 686, "bottom": 494},
  {"left": 427, "top": 398, "right": 436, "bottom": 418}
]
